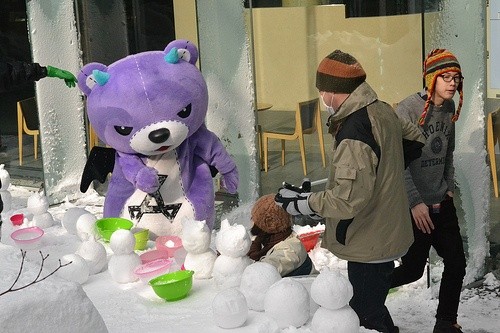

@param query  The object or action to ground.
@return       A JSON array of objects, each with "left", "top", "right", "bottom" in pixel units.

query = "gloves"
[
  {"left": 46, "top": 65, "right": 78, "bottom": 88},
  {"left": 274, "top": 181, "right": 323, "bottom": 220}
]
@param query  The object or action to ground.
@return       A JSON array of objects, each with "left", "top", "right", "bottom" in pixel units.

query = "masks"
[{"left": 320, "top": 94, "right": 335, "bottom": 116}]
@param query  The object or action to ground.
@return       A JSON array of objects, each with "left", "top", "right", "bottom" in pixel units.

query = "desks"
[{"left": 257, "top": 101, "right": 272, "bottom": 172}]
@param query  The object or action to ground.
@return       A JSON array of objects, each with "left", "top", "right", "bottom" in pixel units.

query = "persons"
[
  {"left": 0, "top": 63, "right": 78, "bottom": 88},
  {"left": 247, "top": 194, "right": 320, "bottom": 278},
  {"left": 390, "top": 48, "right": 467, "bottom": 333},
  {"left": 275, "top": 50, "right": 415, "bottom": 333}
]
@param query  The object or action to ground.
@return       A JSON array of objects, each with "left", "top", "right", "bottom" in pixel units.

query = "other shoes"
[{"left": 433, "top": 321, "right": 463, "bottom": 333}]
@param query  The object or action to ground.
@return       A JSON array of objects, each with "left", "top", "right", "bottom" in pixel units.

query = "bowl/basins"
[
  {"left": 133, "top": 258, "right": 172, "bottom": 286},
  {"left": 9, "top": 213, "right": 24, "bottom": 226},
  {"left": 139, "top": 249, "right": 168, "bottom": 264},
  {"left": 297, "top": 229, "right": 325, "bottom": 252},
  {"left": 94, "top": 217, "right": 134, "bottom": 243},
  {"left": 134, "top": 228, "right": 149, "bottom": 250},
  {"left": 10, "top": 226, "right": 44, "bottom": 245},
  {"left": 155, "top": 235, "right": 183, "bottom": 257},
  {"left": 148, "top": 269, "right": 195, "bottom": 302}
]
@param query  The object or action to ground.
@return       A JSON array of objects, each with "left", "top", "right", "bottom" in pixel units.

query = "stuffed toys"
[{"left": 77, "top": 39, "right": 240, "bottom": 232}]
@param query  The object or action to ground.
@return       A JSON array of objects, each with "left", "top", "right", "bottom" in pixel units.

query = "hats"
[
  {"left": 418, "top": 48, "right": 463, "bottom": 126},
  {"left": 252, "top": 194, "right": 290, "bottom": 233},
  {"left": 316, "top": 49, "right": 366, "bottom": 93}
]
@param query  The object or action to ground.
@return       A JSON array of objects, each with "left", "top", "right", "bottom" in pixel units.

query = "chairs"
[
  {"left": 261, "top": 97, "right": 326, "bottom": 177},
  {"left": 17, "top": 97, "right": 40, "bottom": 166},
  {"left": 485, "top": 106, "right": 500, "bottom": 197}
]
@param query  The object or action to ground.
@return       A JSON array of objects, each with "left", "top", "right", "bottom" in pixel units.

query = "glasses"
[{"left": 438, "top": 74, "right": 464, "bottom": 83}]
